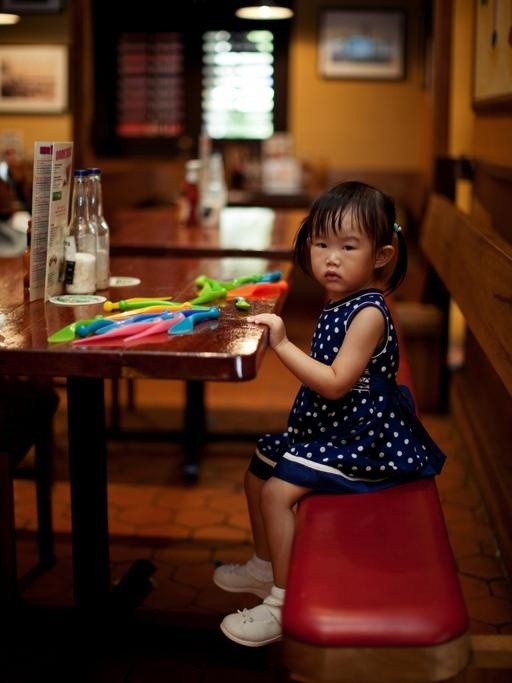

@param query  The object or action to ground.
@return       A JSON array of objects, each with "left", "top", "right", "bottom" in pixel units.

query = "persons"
[{"left": 210, "top": 181, "right": 447, "bottom": 650}]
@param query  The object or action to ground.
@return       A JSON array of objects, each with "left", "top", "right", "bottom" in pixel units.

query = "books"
[{"left": 26, "top": 138, "right": 75, "bottom": 304}]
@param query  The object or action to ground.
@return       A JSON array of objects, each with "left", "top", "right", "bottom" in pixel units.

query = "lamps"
[{"left": 235, "top": 1, "right": 294, "bottom": 21}]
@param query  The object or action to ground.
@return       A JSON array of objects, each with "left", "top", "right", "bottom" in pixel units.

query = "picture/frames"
[{"left": 313, "top": 5, "right": 407, "bottom": 81}]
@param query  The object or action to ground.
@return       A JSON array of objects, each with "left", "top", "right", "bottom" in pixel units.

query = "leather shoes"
[
  {"left": 213, "top": 563, "right": 274, "bottom": 600},
  {"left": 220, "top": 595, "right": 284, "bottom": 648}
]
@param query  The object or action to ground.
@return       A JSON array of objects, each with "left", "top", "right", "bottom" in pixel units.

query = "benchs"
[{"left": 286, "top": 194, "right": 511, "bottom": 681}]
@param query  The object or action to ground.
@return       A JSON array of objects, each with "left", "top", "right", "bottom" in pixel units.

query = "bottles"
[
  {"left": 63, "top": 167, "right": 97, "bottom": 295},
  {"left": 201, "top": 151, "right": 229, "bottom": 227},
  {"left": 87, "top": 169, "right": 111, "bottom": 292},
  {"left": 174, "top": 158, "right": 204, "bottom": 231},
  {"left": 23, "top": 218, "right": 32, "bottom": 295}
]
